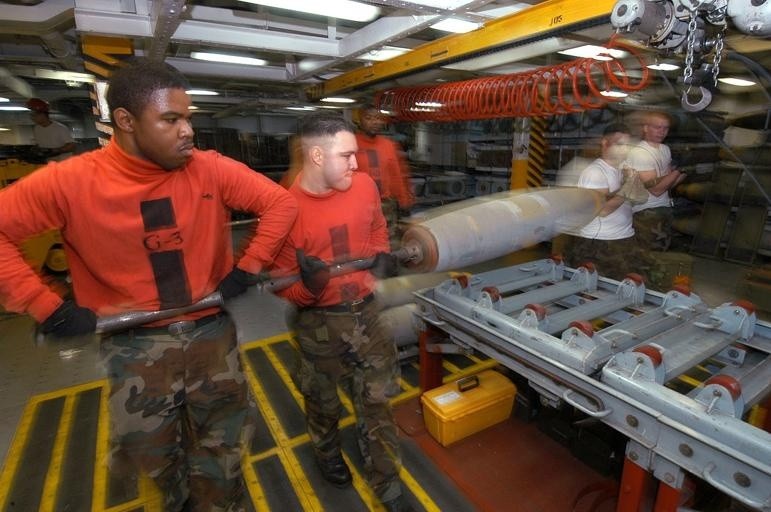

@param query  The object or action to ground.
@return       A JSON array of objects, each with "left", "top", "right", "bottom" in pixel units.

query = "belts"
[
  {"left": 311, "top": 292, "right": 374, "bottom": 313},
  {"left": 127, "top": 311, "right": 225, "bottom": 337}
]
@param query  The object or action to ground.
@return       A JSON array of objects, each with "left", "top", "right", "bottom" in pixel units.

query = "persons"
[
  {"left": 0, "top": 54, "right": 301, "bottom": 511},
  {"left": 353, "top": 103, "right": 419, "bottom": 279},
  {"left": 260, "top": 109, "right": 419, "bottom": 510},
  {"left": 23, "top": 97, "right": 78, "bottom": 168},
  {"left": 558, "top": 122, "right": 647, "bottom": 282},
  {"left": 625, "top": 112, "right": 693, "bottom": 292}
]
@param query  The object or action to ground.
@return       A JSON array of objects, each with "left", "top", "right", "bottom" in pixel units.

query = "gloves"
[
  {"left": 371, "top": 251, "right": 397, "bottom": 278},
  {"left": 39, "top": 300, "right": 95, "bottom": 341},
  {"left": 217, "top": 268, "right": 249, "bottom": 300},
  {"left": 297, "top": 249, "right": 329, "bottom": 295}
]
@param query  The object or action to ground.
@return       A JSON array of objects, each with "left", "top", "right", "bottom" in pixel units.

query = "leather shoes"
[
  {"left": 386, "top": 496, "right": 413, "bottom": 512},
  {"left": 321, "top": 452, "right": 353, "bottom": 488}
]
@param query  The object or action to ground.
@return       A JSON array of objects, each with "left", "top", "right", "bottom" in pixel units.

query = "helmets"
[{"left": 26, "top": 98, "right": 49, "bottom": 112}]
